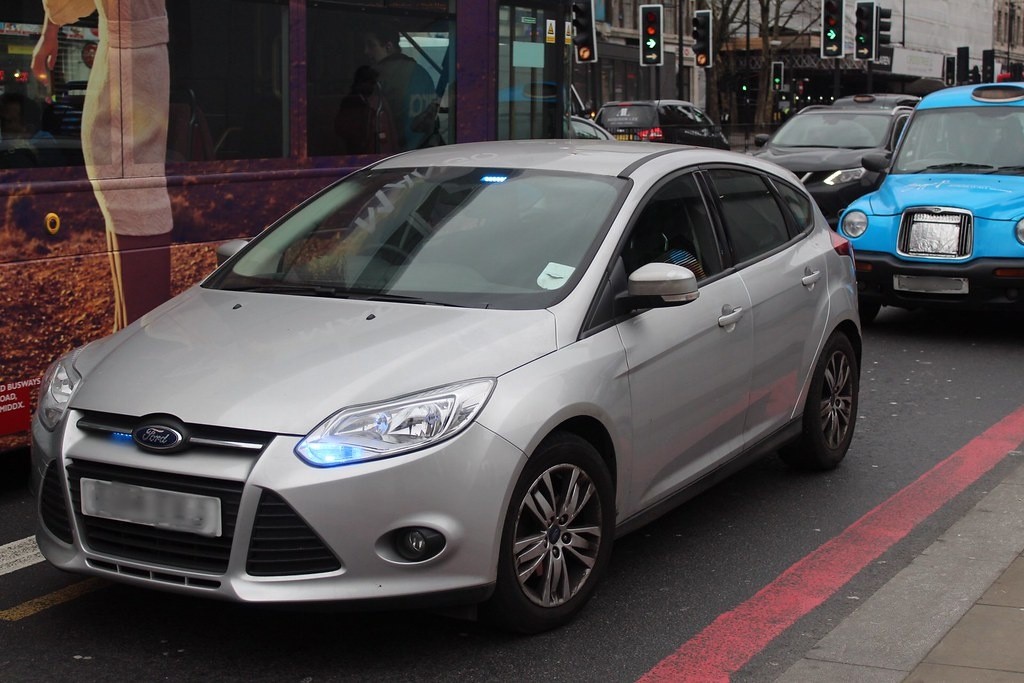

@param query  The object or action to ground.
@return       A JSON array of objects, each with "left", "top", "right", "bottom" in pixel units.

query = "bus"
[{"left": 0, "top": 0, "right": 572, "bottom": 455}]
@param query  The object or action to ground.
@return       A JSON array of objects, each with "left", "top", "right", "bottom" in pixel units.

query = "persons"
[
  {"left": 0, "top": 93, "right": 65, "bottom": 166},
  {"left": 720, "top": 107, "right": 732, "bottom": 139},
  {"left": 170, "top": 67, "right": 215, "bottom": 160},
  {"left": 360, "top": 17, "right": 438, "bottom": 151}
]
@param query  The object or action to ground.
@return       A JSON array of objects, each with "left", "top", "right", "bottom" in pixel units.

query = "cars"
[
  {"left": 837, "top": 82, "right": 1024, "bottom": 324},
  {"left": 30, "top": 139, "right": 864, "bottom": 635},
  {"left": 441, "top": 115, "right": 616, "bottom": 144}
]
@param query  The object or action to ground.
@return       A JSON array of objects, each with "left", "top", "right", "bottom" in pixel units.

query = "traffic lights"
[
  {"left": 820, "top": 0, "right": 845, "bottom": 59},
  {"left": 738, "top": 82, "right": 750, "bottom": 96},
  {"left": 639, "top": 4, "right": 664, "bottom": 67},
  {"left": 770, "top": 61, "right": 784, "bottom": 92},
  {"left": 571, "top": 0, "right": 598, "bottom": 64},
  {"left": 876, "top": 6, "right": 891, "bottom": 60},
  {"left": 692, "top": 10, "right": 713, "bottom": 68},
  {"left": 944, "top": 55, "right": 957, "bottom": 86},
  {"left": 853, "top": 0, "right": 875, "bottom": 61}
]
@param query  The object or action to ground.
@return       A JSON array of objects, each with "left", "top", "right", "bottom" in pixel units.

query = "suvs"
[
  {"left": 594, "top": 99, "right": 730, "bottom": 151},
  {"left": 744, "top": 94, "right": 921, "bottom": 232}
]
